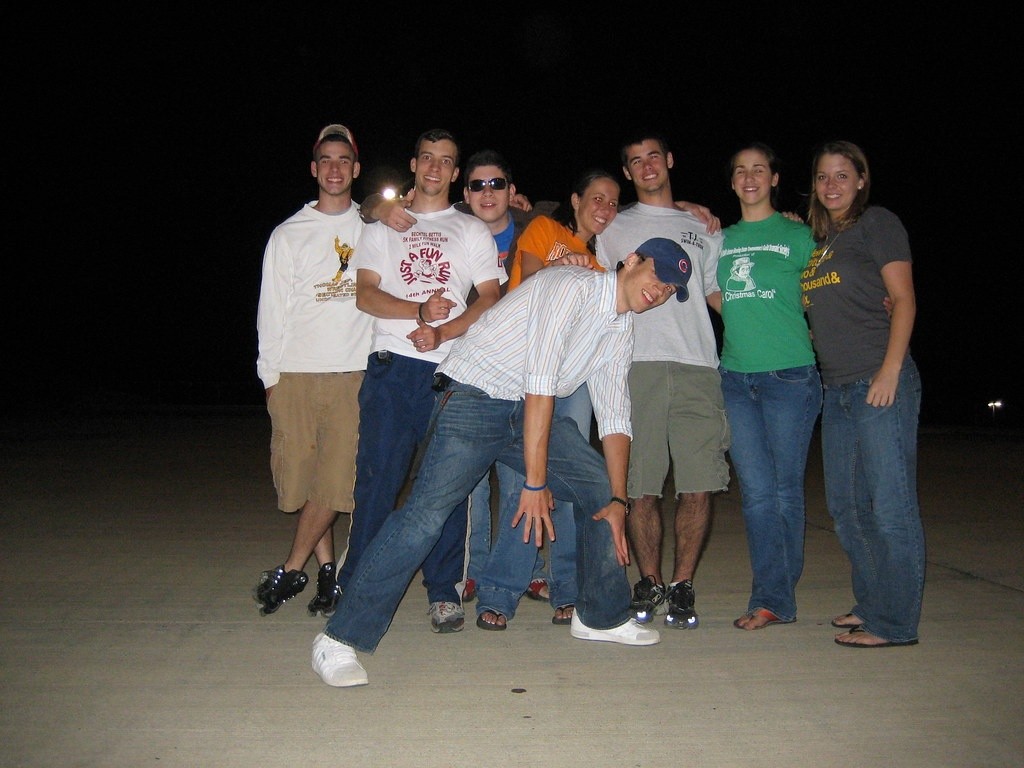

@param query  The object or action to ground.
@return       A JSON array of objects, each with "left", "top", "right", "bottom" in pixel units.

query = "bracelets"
[
  {"left": 419, "top": 303, "right": 425, "bottom": 321},
  {"left": 523, "top": 480, "right": 547, "bottom": 491}
]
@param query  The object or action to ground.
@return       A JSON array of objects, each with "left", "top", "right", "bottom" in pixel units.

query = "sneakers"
[
  {"left": 570, "top": 605, "right": 661, "bottom": 646},
  {"left": 627, "top": 574, "right": 667, "bottom": 623},
  {"left": 310, "top": 632, "right": 368, "bottom": 687},
  {"left": 428, "top": 601, "right": 466, "bottom": 633},
  {"left": 251, "top": 562, "right": 308, "bottom": 617},
  {"left": 306, "top": 562, "right": 342, "bottom": 616},
  {"left": 665, "top": 577, "right": 698, "bottom": 630}
]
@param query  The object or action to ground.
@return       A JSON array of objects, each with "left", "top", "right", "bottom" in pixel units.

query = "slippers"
[
  {"left": 733, "top": 607, "right": 798, "bottom": 629},
  {"left": 835, "top": 625, "right": 918, "bottom": 648},
  {"left": 552, "top": 603, "right": 574, "bottom": 624},
  {"left": 476, "top": 608, "right": 507, "bottom": 631},
  {"left": 461, "top": 578, "right": 478, "bottom": 601},
  {"left": 523, "top": 577, "right": 550, "bottom": 602},
  {"left": 831, "top": 612, "right": 865, "bottom": 628}
]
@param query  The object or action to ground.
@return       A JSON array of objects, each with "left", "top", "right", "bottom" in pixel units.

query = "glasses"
[{"left": 467, "top": 177, "right": 508, "bottom": 192}]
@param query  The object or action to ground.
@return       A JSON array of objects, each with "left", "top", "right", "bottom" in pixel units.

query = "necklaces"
[{"left": 817, "top": 228, "right": 842, "bottom": 264}]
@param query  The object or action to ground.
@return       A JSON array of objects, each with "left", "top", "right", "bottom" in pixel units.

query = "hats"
[
  {"left": 313, "top": 124, "right": 358, "bottom": 161},
  {"left": 635, "top": 237, "right": 692, "bottom": 303}
]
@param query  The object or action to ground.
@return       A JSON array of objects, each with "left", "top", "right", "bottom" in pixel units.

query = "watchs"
[{"left": 611, "top": 497, "right": 631, "bottom": 516}]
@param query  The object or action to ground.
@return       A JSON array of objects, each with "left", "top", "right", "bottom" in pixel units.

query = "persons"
[
  {"left": 477, "top": 166, "right": 621, "bottom": 631},
  {"left": 359, "top": 153, "right": 560, "bottom": 602},
  {"left": 311, "top": 238, "right": 692, "bottom": 686},
  {"left": 717, "top": 139, "right": 893, "bottom": 631},
  {"left": 318, "top": 118, "right": 510, "bottom": 633},
  {"left": 594, "top": 141, "right": 806, "bottom": 630},
  {"left": 784, "top": 140, "right": 925, "bottom": 648},
  {"left": 251, "top": 124, "right": 361, "bottom": 617}
]
